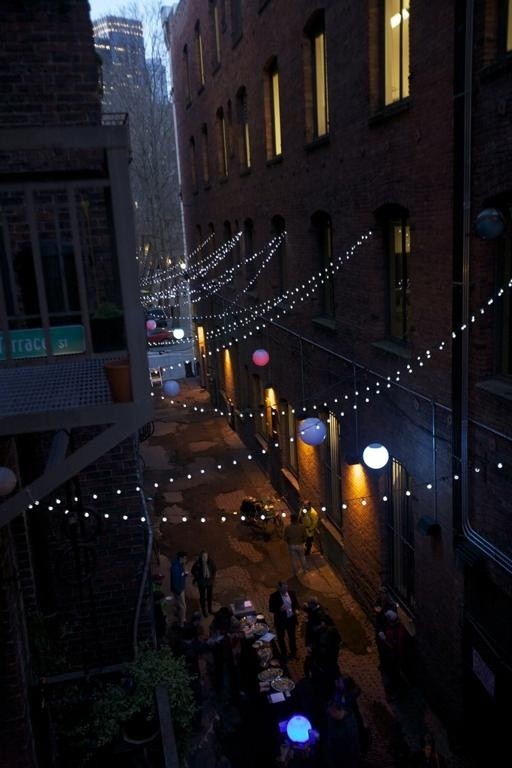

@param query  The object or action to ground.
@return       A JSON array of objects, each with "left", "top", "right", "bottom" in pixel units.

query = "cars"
[{"left": 148, "top": 327, "right": 176, "bottom": 346}]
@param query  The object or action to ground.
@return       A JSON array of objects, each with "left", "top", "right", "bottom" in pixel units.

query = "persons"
[
  {"left": 191, "top": 550, "right": 216, "bottom": 617},
  {"left": 170, "top": 552, "right": 190, "bottom": 628},
  {"left": 297, "top": 499, "right": 320, "bottom": 559},
  {"left": 269, "top": 581, "right": 302, "bottom": 661},
  {"left": 417, "top": 740, "right": 436, "bottom": 768},
  {"left": 284, "top": 513, "right": 310, "bottom": 578},
  {"left": 153, "top": 588, "right": 365, "bottom": 768}
]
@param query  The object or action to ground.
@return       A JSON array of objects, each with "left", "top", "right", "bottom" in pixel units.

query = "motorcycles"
[{"left": 240, "top": 496, "right": 282, "bottom": 542}]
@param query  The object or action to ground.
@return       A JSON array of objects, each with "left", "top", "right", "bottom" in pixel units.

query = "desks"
[{"left": 227, "top": 600, "right": 320, "bottom": 767}]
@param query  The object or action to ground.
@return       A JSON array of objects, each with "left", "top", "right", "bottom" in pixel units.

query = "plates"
[
  {"left": 257, "top": 667, "right": 282, "bottom": 682},
  {"left": 270, "top": 677, "right": 296, "bottom": 692}
]
